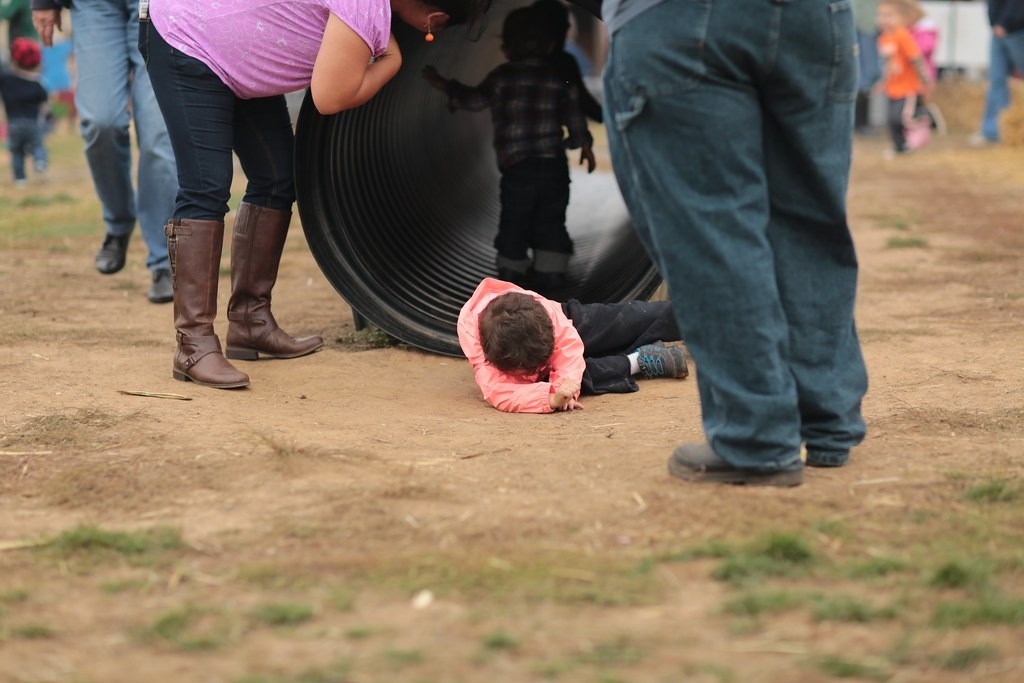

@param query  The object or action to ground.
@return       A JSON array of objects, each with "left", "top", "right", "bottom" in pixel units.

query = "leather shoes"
[
  {"left": 152, "top": 269, "right": 176, "bottom": 303},
  {"left": 666, "top": 439, "right": 800, "bottom": 488},
  {"left": 95, "top": 228, "right": 129, "bottom": 274},
  {"left": 806, "top": 441, "right": 848, "bottom": 468}
]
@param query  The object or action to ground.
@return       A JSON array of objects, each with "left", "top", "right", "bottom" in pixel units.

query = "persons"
[
  {"left": 0, "top": 38, "right": 54, "bottom": 184},
  {"left": 138, "top": 0, "right": 489, "bottom": 389},
  {"left": 875, "top": 1, "right": 946, "bottom": 153},
  {"left": 29, "top": 0, "right": 180, "bottom": 304},
  {"left": 967, "top": 0, "right": 1024, "bottom": 147},
  {"left": 423, "top": 1, "right": 606, "bottom": 274},
  {"left": 457, "top": 276, "right": 688, "bottom": 413},
  {"left": 601, "top": 0, "right": 869, "bottom": 488}
]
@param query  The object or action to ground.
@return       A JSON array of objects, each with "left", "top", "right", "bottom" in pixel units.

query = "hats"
[{"left": 11, "top": 36, "right": 41, "bottom": 64}]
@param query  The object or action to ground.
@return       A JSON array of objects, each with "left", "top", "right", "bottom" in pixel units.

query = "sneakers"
[{"left": 636, "top": 338, "right": 689, "bottom": 378}]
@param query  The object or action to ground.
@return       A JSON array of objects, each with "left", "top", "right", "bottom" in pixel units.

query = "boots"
[
  {"left": 223, "top": 201, "right": 324, "bottom": 358},
  {"left": 165, "top": 217, "right": 250, "bottom": 388}
]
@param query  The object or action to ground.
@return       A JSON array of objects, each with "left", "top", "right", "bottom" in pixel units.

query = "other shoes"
[
  {"left": 496, "top": 248, "right": 584, "bottom": 295},
  {"left": 13, "top": 178, "right": 27, "bottom": 190},
  {"left": 33, "top": 161, "right": 45, "bottom": 173},
  {"left": 966, "top": 134, "right": 989, "bottom": 145}
]
